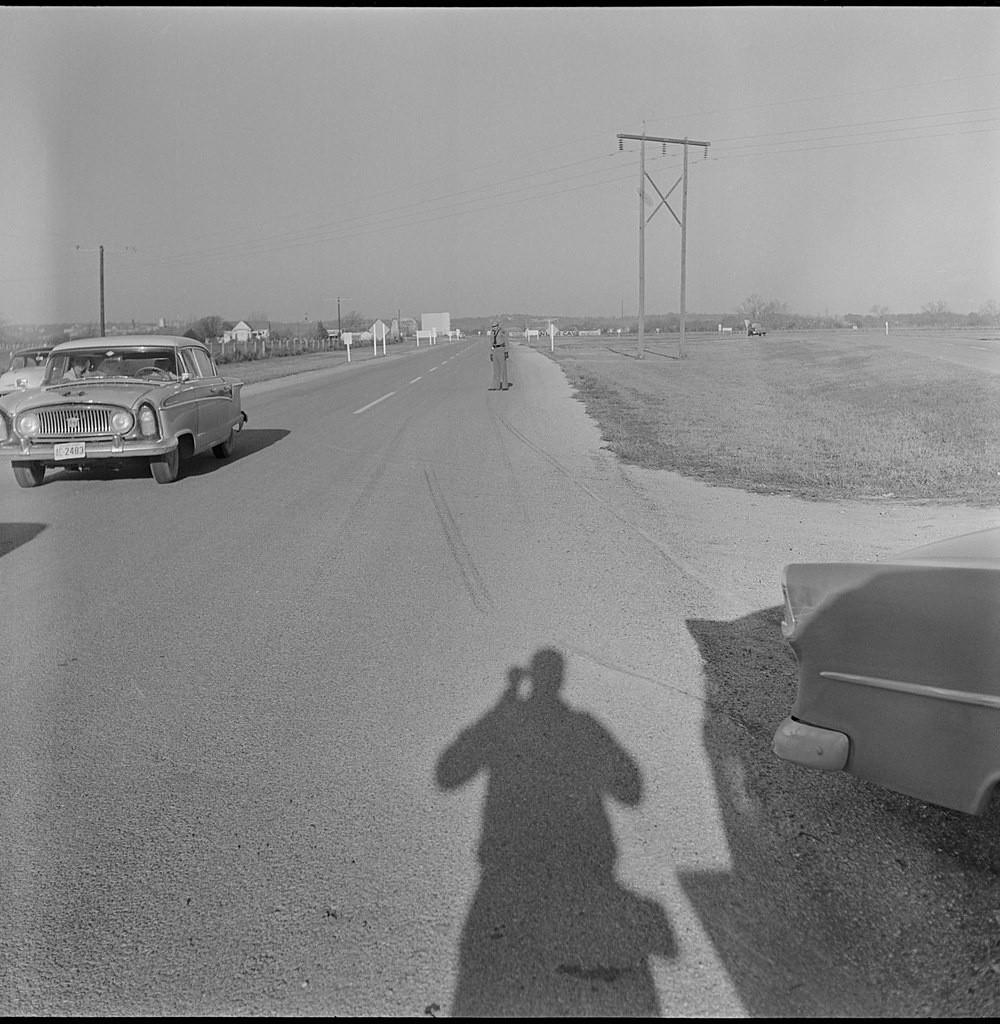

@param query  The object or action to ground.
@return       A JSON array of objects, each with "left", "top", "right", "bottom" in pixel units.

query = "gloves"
[
  {"left": 504, "top": 351, "right": 508, "bottom": 360},
  {"left": 490, "top": 354, "right": 493, "bottom": 361}
]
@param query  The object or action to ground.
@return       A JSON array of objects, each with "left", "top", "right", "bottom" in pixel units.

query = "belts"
[{"left": 493, "top": 344, "right": 505, "bottom": 347}]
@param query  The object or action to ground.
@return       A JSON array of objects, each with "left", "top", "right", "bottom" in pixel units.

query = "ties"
[{"left": 493, "top": 328, "right": 501, "bottom": 344}]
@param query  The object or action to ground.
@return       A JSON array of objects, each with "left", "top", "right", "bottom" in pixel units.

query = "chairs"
[{"left": 154, "top": 359, "right": 181, "bottom": 376}]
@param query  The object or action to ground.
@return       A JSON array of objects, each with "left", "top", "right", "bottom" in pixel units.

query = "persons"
[
  {"left": 488, "top": 321, "right": 510, "bottom": 390},
  {"left": 62, "top": 356, "right": 106, "bottom": 380},
  {"left": 149, "top": 359, "right": 180, "bottom": 381}
]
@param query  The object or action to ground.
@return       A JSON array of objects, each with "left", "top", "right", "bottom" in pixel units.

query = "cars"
[
  {"left": 748, "top": 321, "right": 767, "bottom": 339},
  {"left": 0, "top": 333, "right": 246, "bottom": 487},
  {"left": 0, "top": 346, "right": 65, "bottom": 395}
]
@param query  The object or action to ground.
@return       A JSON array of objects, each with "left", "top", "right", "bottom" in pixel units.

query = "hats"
[{"left": 492, "top": 323, "right": 499, "bottom": 327}]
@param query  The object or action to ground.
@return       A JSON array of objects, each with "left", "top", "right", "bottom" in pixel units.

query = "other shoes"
[
  {"left": 489, "top": 388, "right": 496, "bottom": 390},
  {"left": 503, "top": 388, "right": 508, "bottom": 390}
]
